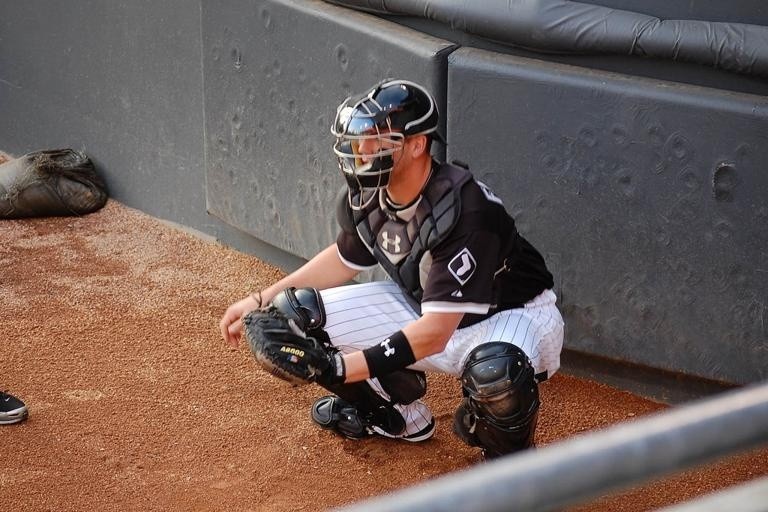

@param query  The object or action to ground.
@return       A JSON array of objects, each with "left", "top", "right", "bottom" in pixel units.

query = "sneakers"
[{"left": 312, "top": 395, "right": 438, "bottom": 443}]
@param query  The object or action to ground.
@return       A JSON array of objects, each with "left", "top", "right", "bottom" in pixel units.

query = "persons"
[{"left": 219, "top": 78, "right": 565, "bottom": 463}]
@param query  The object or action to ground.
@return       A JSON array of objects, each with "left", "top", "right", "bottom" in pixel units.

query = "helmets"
[{"left": 331, "top": 77, "right": 448, "bottom": 147}]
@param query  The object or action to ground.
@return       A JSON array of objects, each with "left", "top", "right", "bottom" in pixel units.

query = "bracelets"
[{"left": 362, "top": 330, "right": 417, "bottom": 379}]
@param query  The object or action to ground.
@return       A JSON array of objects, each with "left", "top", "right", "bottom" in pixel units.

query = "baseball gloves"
[{"left": 244, "top": 308, "right": 345, "bottom": 388}]
